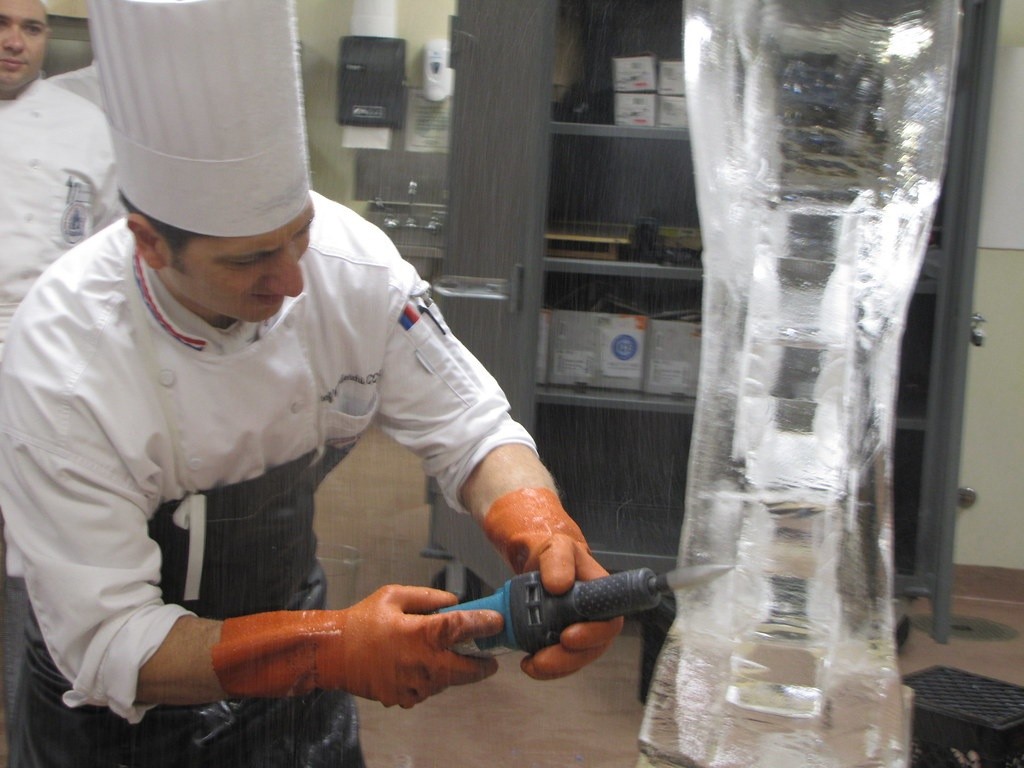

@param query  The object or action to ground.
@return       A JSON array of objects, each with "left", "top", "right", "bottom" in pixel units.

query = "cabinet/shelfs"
[{"left": 422, "top": 0, "right": 1003, "bottom": 646}]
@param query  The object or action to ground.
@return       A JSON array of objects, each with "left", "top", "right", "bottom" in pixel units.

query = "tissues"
[{"left": 334, "top": 36, "right": 406, "bottom": 151}]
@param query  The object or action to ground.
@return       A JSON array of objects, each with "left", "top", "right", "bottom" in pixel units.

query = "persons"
[
  {"left": 0, "top": 0, "right": 621, "bottom": 767},
  {"left": 0, "top": 0, "right": 129, "bottom": 357}
]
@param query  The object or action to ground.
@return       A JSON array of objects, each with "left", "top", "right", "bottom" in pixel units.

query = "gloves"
[
  {"left": 211, "top": 581, "right": 508, "bottom": 709},
  {"left": 482, "top": 488, "right": 626, "bottom": 683}
]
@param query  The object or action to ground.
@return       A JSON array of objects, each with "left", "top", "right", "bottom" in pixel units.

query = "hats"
[{"left": 88, "top": 1, "right": 312, "bottom": 238}]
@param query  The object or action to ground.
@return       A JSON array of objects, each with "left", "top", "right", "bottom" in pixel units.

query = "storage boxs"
[
  {"left": 611, "top": 54, "right": 658, "bottom": 92},
  {"left": 656, "top": 94, "right": 687, "bottom": 129},
  {"left": 902, "top": 663, "right": 1024, "bottom": 768},
  {"left": 537, "top": 300, "right": 701, "bottom": 398},
  {"left": 613, "top": 92, "right": 656, "bottom": 125},
  {"left": 658, "top": 61, "right": 684, "bottom": 96}
]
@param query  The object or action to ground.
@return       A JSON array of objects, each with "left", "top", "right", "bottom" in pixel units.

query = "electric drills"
[{"left": 424, "top": 563, "right": 739, "bottom": 659}]
